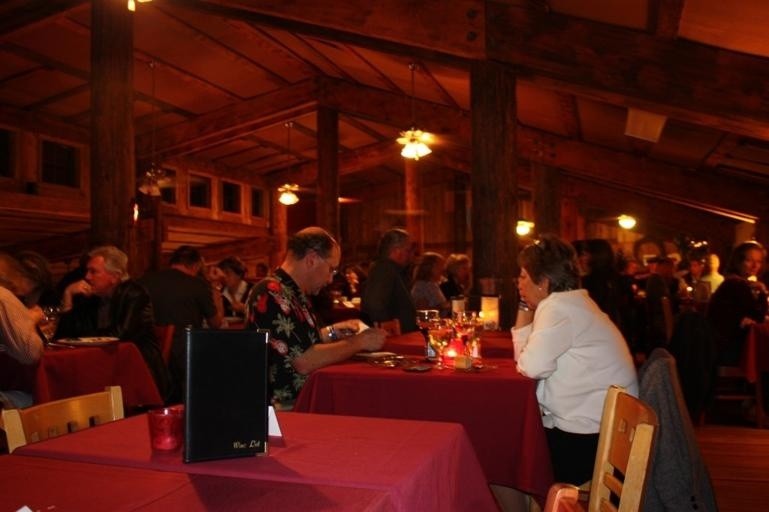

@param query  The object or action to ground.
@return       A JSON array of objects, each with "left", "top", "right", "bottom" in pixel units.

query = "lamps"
[
  {"left": 137, "top": 62, "right": 166, "bottom": 197},
  {"left": 280, "top": 126, "right": 299, "bottom": 206},
  {"left": 395, "top": 59, "right": 439, "bottom": 161}
]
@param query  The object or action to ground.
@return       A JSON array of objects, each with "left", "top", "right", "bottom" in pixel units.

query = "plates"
[
  {"left": 59, "top": 337, "right": 120, "bottom": 346},
  {"left": 358, "top": 350, "right": 431, "bottom": 371}
]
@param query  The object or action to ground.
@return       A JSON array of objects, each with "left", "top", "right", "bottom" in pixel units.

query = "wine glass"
[
  {"left": 36, "top": 307, "right": 60, "bottom": 353},
  {"left": 417, "top": 309, "right": 482, "bottom": 369}
]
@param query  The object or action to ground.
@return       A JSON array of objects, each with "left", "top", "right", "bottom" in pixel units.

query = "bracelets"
[
  {"left": 518, "top": 299, "right": 536, "bottom": 312},
  {"left": 327, "top": 324, "right": 338, "bottom": 341}
]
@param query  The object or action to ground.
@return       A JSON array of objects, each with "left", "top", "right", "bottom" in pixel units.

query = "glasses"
[{"left": 320, "top": 255, "right": 339, "bottom": 274}]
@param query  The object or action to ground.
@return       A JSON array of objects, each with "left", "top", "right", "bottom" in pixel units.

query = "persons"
[
  {"left": 0, "top": 283, "right": 47, "bottom": 409},
  {"left": 42, "top": 232, "right": 268, "bottom": 408},
  {"left": 565, "top": 233, "right": 769, "bottom": 408},
  {"left": 308, "top": 232, "right": 520, "bottom": 342},
  {"left": 361, "top": 227, "right": 419, "bottom": 334},
  {"left": 510, "top": 235, "right": 640, "bottom": 489},
  {"left": 243, "top": 226, "right": 391, "bottom": 412}
]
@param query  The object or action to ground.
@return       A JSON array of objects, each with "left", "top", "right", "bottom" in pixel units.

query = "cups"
[{"left": 146, "top": 408, "right": 185, "bottom": 452}]
